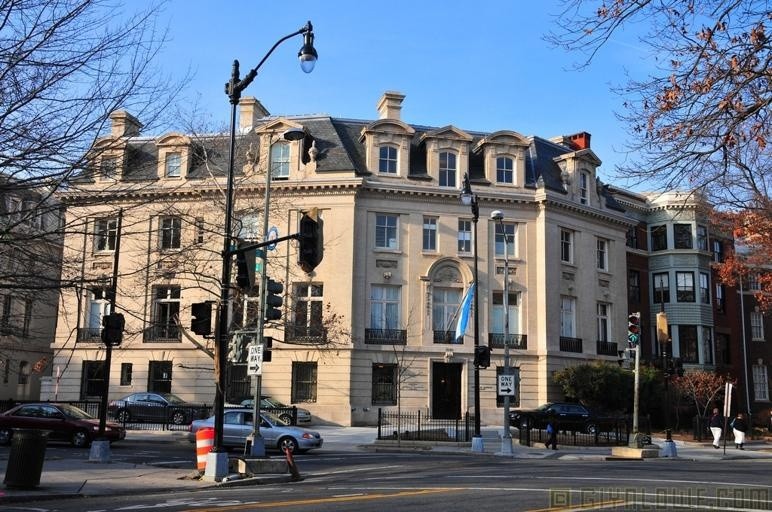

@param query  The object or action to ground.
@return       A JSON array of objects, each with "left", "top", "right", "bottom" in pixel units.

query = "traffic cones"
[{"left": 284, "top": 447, "right": 306, "bottom": 483}]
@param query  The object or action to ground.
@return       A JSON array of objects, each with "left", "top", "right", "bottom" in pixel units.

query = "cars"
[
  {"left": 504, "top": 402, "right": 615, "bottom": 435},
  {"left": 0, "top": 402, "right": 126, "bottom": 450},
  {"left": 106, "top": 390, "right": 325, "bottom": 456}
]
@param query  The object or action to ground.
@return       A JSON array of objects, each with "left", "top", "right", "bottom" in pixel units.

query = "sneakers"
[
  {"left": 736, "top": 446, "right": 745, "bottom": 450},
  {"left": 713, "top": 445, "right": 720, "bottom": 449},
  {"left": 543, "top": 443, "right": 558, "bottom": 450}
]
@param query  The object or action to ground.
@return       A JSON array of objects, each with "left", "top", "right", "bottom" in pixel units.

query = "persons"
[
  {"left": 766, "top": 409, "right": 772, "bottom": 432},
  {"left": 732, "top": 413, "right": 746, "bottom": 449},
  {"left": 544, "top": 409, "right": 559, "bottom": 450},
  {"left": 709, "top": 408, "right": 723, "bottom": 448}
]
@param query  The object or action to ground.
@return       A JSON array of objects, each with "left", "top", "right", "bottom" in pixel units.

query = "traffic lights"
[
  {"left": 265, "top": 280, "right": 285, "bottom": 321},
  {"left": 627, "top": 313, "right": 641, "bottom": 348}
]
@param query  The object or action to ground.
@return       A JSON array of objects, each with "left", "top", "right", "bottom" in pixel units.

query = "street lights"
[
  {"left": 488, "top": 207, "right": 512, "bottom": 460},
  {"left": 458, "top": 173, "right": 489, "bottom": 450}
]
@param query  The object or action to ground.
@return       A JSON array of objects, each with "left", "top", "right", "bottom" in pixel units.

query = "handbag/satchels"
[
  {"left": 730, "top": 421, "right": 735, "bottom": 428},
  {"left": 545, "top": 424, "right": 553, "bottom": 434}
]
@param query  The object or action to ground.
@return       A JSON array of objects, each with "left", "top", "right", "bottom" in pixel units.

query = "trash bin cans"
[{"left": 4, "top": 428, "right": 52, "bottom": 490}]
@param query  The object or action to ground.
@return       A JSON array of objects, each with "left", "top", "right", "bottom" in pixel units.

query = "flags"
[{"left": 454, "top": 281, "right": 475, "bottom": 340}]
